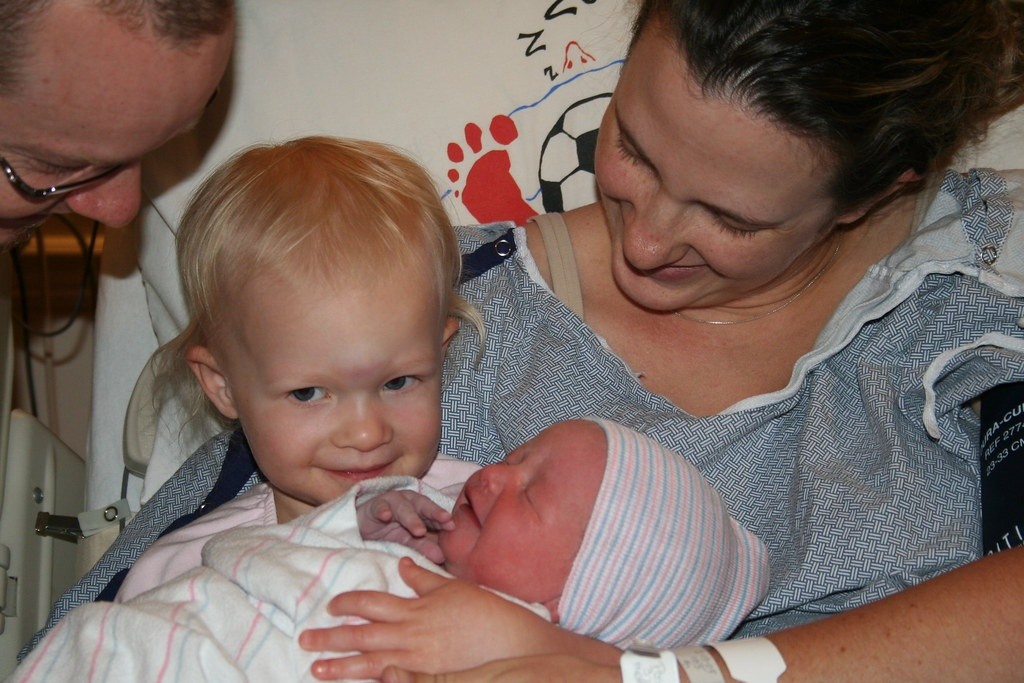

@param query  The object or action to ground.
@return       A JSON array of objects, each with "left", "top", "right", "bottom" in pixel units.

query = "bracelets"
[{"left": 618, "top": 635, "right": 787, "bottom": 683}]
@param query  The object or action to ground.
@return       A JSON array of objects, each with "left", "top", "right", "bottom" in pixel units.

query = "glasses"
[{"left": 0, "top": 153, "right": 124, "bottom": 202}]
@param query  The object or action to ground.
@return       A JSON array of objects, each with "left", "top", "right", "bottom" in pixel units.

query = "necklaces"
[{"left": 676, "top": 233, "right": 841, "bottom": 326}]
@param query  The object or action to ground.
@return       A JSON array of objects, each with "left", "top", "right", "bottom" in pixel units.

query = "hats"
[{"left": 554, "top": 414, "right": 772, "bottom": 654}]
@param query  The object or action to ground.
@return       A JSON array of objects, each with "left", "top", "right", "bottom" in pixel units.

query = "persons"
[
  {"left": 0, "top": 0, "right": 235, "bottom": 229},
  {"left": 11, "top": 0, "right": 1024, "bottom": 683}
]
